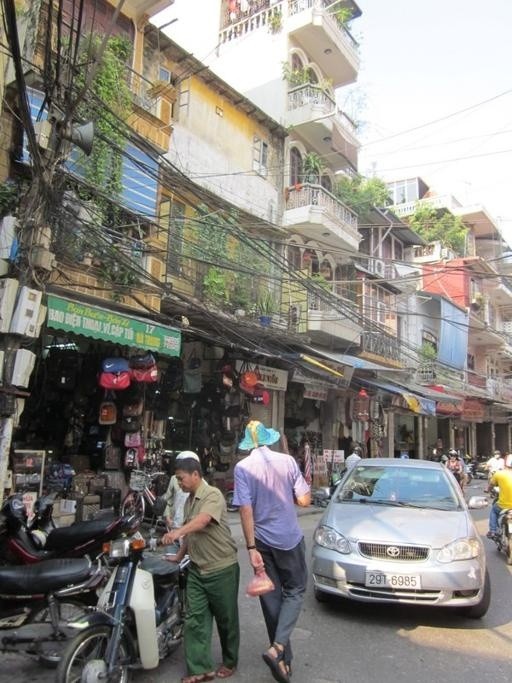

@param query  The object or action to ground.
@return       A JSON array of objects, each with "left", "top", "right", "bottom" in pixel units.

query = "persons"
[
  {"left": 344, "top": 446, "right": 363, "bottom": 472},
  {"left": 163, "top": 449, "right": 207, "bottom": 556},
  {"left": 426, "top": 435, "right": 512, "bottom": 541},
  {"left": 163, "top": 456, "right": 241, "bottom": 683},
  {"left": 231, "top": 420, "right": 313, "bottom": 683}
]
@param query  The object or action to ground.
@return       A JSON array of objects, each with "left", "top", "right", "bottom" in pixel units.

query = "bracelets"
[{"left": 246, "top": 543, "right": 257, "bottom": 550}]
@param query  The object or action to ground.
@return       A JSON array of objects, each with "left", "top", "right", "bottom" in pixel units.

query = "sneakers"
[{"left": 486, "top": 531, "right": 497, "bottom": 538}]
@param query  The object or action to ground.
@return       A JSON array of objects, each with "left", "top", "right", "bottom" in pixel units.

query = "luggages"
[
  {"left": 78, "top": 494, "right": 100, "bottom": 521},
  {"left": 102, "top": 486, "right": 121, "bottom": 515}
]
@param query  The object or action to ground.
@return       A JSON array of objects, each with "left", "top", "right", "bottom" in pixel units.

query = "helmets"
[
  {"left": 449, "top": 450, "right": 458, "bottom": 456},
  {"left": 354, "top": 446, "right": 362, "bottom": 452},
  {"left": 504, "top": 454, "right": 512, "bottom": 467}
]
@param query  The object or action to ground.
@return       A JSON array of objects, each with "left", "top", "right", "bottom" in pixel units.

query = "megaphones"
[{"left": 62, "top": 121, "right": 94, "bottom": 156}]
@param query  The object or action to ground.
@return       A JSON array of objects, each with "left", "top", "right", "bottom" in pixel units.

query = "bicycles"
[
  {"left": 222, "top": 482, "right": 241, "bottom": 512},
  {"left": 117, "top": 464, "right": 167, "bottom": 527}
]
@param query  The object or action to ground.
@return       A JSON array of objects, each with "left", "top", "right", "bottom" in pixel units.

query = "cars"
[{"left": 303, "top": 453, "right": 495, "bottom": 621}]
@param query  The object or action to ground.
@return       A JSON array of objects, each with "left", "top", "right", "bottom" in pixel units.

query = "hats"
[{"left": 238, "top": 419, "right": 281, "bottom": 450}]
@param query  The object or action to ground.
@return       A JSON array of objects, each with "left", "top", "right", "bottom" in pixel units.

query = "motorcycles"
[
  {"left": 439, "top": 454, "right": 512, "bottom": 565},
  {"left": 0, "top": 482, "right": 189, "bottom": 681}
]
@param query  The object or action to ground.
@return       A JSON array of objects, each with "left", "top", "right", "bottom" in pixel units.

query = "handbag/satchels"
[
  {"left": 100, "top": 354, "right": 158, "bottom": 389},
  {"left": 103, "top": 443, "right": 122, "bottom": 471},
  {"left": 18, "top": 348, "right": 98, "bottom": 432},
  {"left": 66, "top": 469, "right": 112, "bottom": 501},
  {"left": 154, "top": 369, "right": 241, "bottom": 476},
  {"left": 99, "top": 401, "right": 117, "bottom": 426},
  {"left": 239, "top": 369, "right": 271, "bottom": 421},
  {"left": 123, "top": 398, "right": 145, "bottom": 470}
]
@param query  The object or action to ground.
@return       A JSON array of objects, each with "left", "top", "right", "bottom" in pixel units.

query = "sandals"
[
  {"left": 263, "top": 644, "right": 290, "bottom": 682},
  {"left": 182, "top": 672, "right": 213, "bottom": 682},
  {"left": 218, "top": 666, "right": 236, "bottom": 678}
]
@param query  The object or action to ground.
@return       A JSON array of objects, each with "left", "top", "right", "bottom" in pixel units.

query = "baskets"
[{"left": 130, "top": 472, "right": 146, "bottom": 492}]
